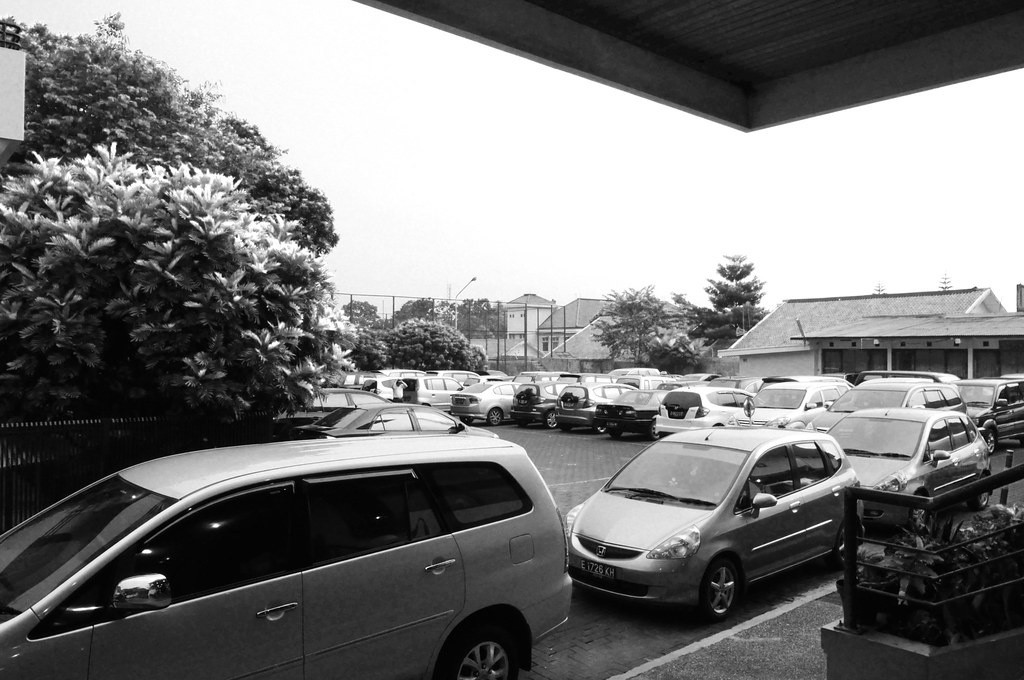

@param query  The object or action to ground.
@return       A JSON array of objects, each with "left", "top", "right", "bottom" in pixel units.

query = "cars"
[
  {"left": 0, "top": 433, "right": 572, "bottom": 680},
  {"left": 271, "top": 369, "right": 1024, "bottom": 442},
  {"left": 564, "top": 426, "right": 861, "bottom": 620},
  {"left": 823, "top": 408, "right": 993, "bottom": 534}
]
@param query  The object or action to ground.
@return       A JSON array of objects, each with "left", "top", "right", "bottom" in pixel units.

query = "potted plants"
[{"left": 836, "top": 503, "right": 1024, "bottom": 641}]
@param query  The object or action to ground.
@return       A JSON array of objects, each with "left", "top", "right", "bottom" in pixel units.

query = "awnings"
[{"left": 352, "top": 0, "right": 1024, "bottom": 133}]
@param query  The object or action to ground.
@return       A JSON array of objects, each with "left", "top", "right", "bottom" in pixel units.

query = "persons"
[{"left": 392, "top": 378, "right": 408, "bottom": 404}]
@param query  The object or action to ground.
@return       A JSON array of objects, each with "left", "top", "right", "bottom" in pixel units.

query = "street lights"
[{"left": 454, "top": 277, "right": 477, "bottom": 329}]
